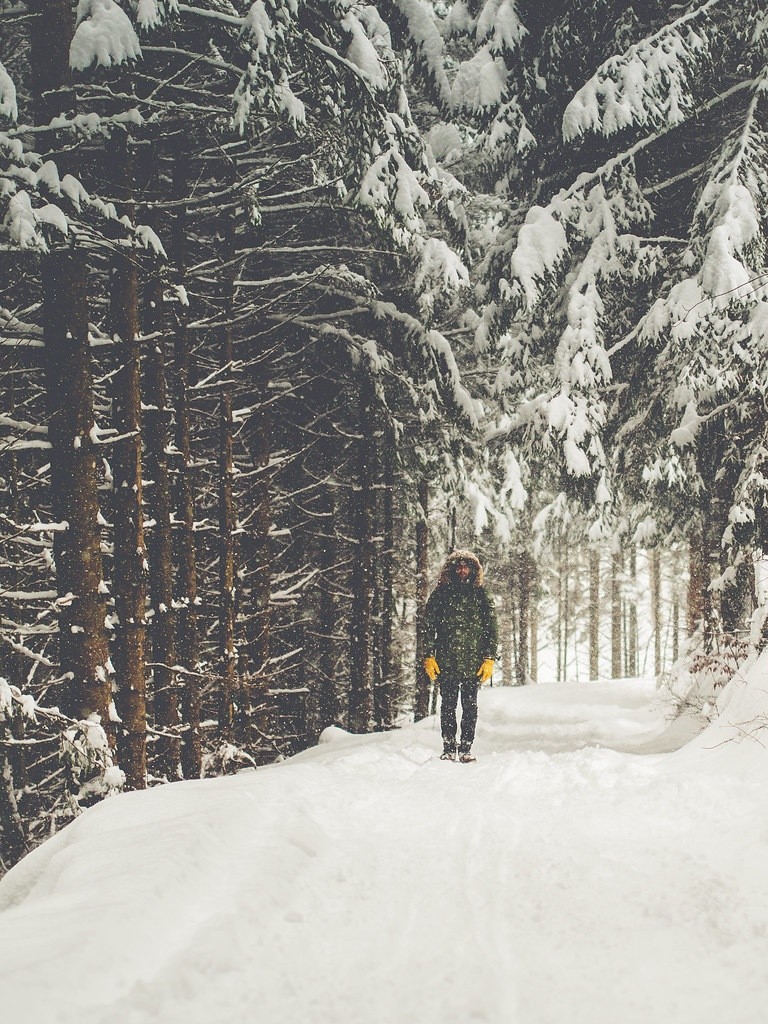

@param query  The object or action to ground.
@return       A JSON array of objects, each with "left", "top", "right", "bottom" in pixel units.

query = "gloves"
[
  {"left": 477, "top": 659, "right": 494, "bottom": 684},
  {"left": 424, "top": 657, "right": 440, "bottom": 680}
]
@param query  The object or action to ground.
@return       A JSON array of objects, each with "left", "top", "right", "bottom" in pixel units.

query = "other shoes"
[
  {"left": 441, "top": 753, "right": 456, "bottom": 762},
  {"left": 459, "top": 752, "right": 476, "bottom": 763}
]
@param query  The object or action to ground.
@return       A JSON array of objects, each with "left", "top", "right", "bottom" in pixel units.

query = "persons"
[{"left": 422, "top": 550, "right": 496, "bottom": 763}]
